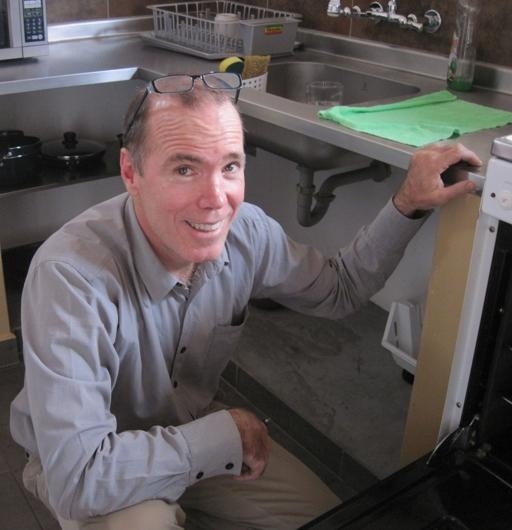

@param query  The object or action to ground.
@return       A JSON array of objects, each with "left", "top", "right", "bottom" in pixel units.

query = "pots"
[
  {"left": 0, "top": 130, "right": 40, "bottom": 193},
  {"left": 37, "top": 131, "right": 106, "bottom": 184}
]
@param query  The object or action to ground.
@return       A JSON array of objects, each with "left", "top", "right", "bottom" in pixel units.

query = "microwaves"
[{"left": 0, "top": 0, "right": 49, "bottom": 61}]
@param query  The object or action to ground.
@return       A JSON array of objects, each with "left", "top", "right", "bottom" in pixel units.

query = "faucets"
[{"left": 327, "top": 0, "right": 423, "bottom": 33}]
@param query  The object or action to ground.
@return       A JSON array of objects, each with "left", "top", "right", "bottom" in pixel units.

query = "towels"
[{"left": 318, "top": 90, "right": 511, "bottom": 147}]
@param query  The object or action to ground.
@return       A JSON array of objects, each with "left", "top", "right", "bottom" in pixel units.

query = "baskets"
[{"left": 146, "top": 0, "right": 301, "bottom": 53}]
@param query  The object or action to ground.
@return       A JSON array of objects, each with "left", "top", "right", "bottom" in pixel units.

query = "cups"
[
  {"left": 307, "top": 80, "right": 341, "bottom": 109},
  {"left": 216, "top": 11, "right": 238, "bottom": 40}
]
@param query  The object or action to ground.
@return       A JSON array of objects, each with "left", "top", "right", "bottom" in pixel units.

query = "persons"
[{"left": 7, "top": 72, "right": 484, "bottom": 530}]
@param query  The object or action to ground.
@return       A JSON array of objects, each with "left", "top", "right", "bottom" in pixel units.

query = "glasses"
[{"left": 123, "top": 72, "right": 242, "bottom": 138}]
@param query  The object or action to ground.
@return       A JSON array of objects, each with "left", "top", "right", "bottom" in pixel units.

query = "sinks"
[{"left": 222, "top": 60, "right": 422, "bottom": 112}]
